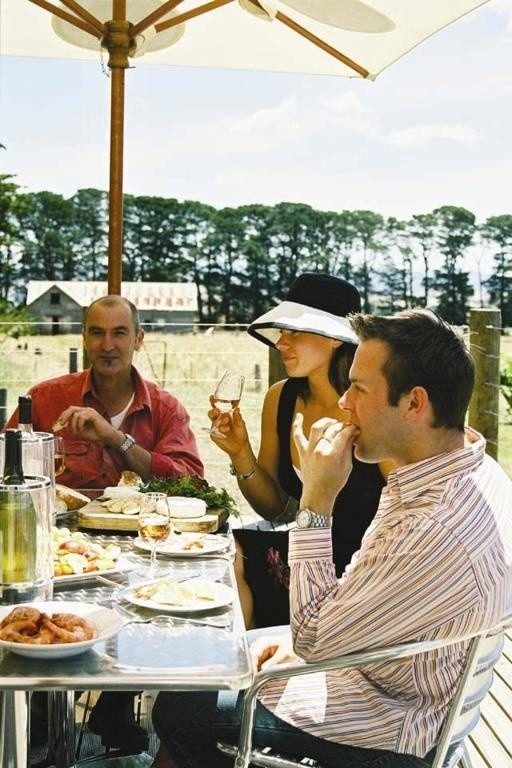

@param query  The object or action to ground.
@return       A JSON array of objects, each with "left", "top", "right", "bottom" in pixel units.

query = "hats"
[{"left": 248, "top": 272, "right": 362, "bottom": 349}]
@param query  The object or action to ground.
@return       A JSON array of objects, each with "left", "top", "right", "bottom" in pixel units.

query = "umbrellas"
[{"left": 1, "top": 2, "right": 490, "bottom": 296}]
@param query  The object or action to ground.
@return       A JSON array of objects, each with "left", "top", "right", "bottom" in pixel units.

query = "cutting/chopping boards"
[{"left": 76, "top": 489, "right": 234, "bottom": 534}]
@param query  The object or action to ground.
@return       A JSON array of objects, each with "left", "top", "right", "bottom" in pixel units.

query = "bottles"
[
  {"left": 2, "top": 387, "right": 37, "bottom": 434},
  {"left": 0, "top": 425, "right": 41, "bottom": 604}
]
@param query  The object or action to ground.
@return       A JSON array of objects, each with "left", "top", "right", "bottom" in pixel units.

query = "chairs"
[{"left": 215, "top": 617, "right": 512, "bottom": 768}]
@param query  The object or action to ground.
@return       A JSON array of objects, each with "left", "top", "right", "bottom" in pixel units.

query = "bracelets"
[{"left": 230, "top": 458, "right": 258, "bottom": 480}]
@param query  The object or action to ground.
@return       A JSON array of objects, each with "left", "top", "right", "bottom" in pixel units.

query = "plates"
[
  {"left": 95, "top": 616, "right": 238, "bottom": 676},
  {"left": 118, "top": 577, "right": 235, "bottom": 614},
  {"left": 50, "top": 529, "right": 135, "bottom": 586},
  {"left": 0, "top": 601, "right": 130, "bottom": 663},
  {"left": 135, "top": 530, "right": 235, "bottom": 559}
]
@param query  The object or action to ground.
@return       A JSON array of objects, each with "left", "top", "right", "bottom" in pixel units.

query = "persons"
[
  {"left": 0, "top": 295, "right": 205, "bottom": 754},
  {"left": 149, "top": 311, "right": 512, "bottom": 767},
  {"left": 206, "top": 272, "right": 397, "bottom": 630}
]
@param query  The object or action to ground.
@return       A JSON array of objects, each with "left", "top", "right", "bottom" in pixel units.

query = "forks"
[{"left": 110, "top": 601, "right": 230, "bottom": 629}]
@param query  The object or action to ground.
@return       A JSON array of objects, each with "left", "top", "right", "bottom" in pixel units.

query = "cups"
[{"left": 0, "top": 430, "right": 61, "bottom": 522}]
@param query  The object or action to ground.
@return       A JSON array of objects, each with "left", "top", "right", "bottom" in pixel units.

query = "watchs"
[
  {"left": 118, "top": 432, "right": 134, "bottom": 451},
  {"left": 295, "top": 505, "right": 333, "bottom": 528}
]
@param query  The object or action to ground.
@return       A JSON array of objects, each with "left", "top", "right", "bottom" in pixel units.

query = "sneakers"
[{"left": 87, "top": 693, "right": 150, "bottom": 751}]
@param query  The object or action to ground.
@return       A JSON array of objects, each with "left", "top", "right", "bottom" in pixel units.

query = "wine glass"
[
  {"left": 202, "top": 371, "right": 246, "bottom": 440},
  {"left": 138, "top": 490, "right": 171, "bottom": 578}
]
